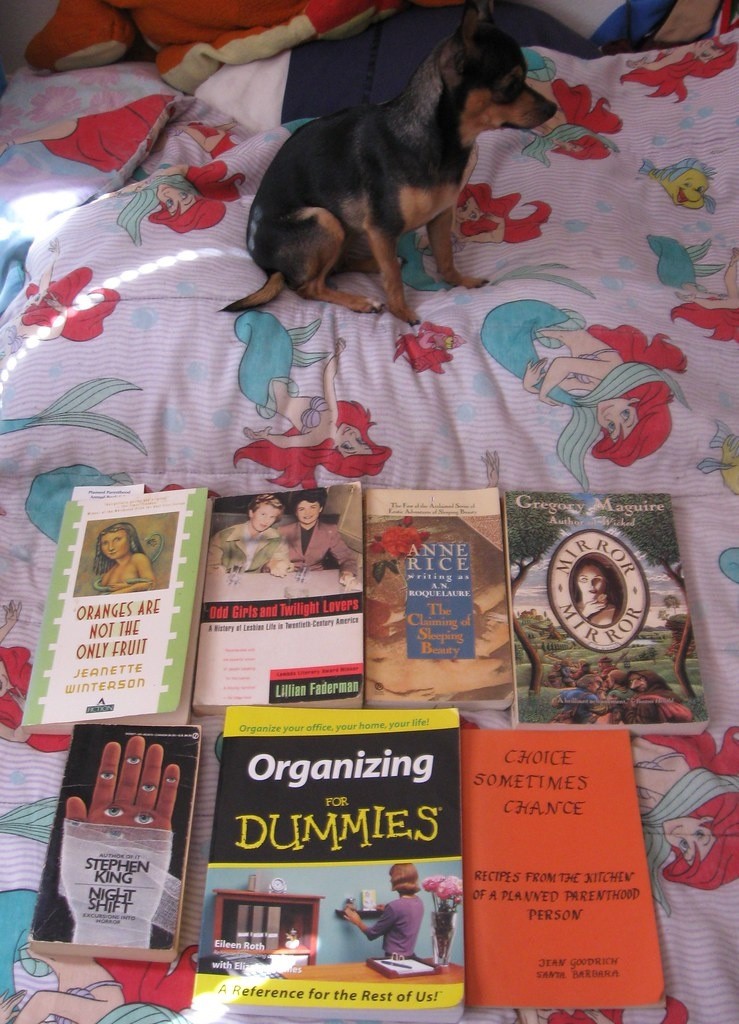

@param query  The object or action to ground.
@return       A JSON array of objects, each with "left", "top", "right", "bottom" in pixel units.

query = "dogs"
[{"left": 218, "top": 0, "right": 558, "bottom": 328}]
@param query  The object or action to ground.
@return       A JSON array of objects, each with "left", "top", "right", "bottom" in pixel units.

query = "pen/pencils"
[{"left": 381, "top": 960, "right": 411, "bottom": 970}]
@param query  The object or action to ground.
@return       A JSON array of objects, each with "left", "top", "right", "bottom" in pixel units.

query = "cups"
[
  {"left": 295, "top": 563, "right": 310, "bottom": 584},
  {"left": 227, "top": 558, "right": 247, "bottom": 584}
]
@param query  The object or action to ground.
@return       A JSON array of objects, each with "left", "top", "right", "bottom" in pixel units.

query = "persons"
[
  {"left": 344, "top": 863, "right": 424, "bottom": 959},
  {"left": 276, "top": 487, "right": 358, "bottom": 585},
  {"left": 205, "top": 495, "right": 290, "bottom": 578}
]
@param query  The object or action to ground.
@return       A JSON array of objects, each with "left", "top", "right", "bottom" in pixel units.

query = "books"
[
  {"left": 459, "top": 729, "right": 667, "bottom": 1010},
  {"left": 503, "top": 492, "right": 709, "bottom": 735},
  {"left": 192, "top": 481, "right": 363, "bottom": 718},
  {"left": 190, "top": 705, "right": 465, "bottom": 1024},
  {"left": 20, "top": 487, "right": 212, "bottom": 735},
  {"left": 363, "top": 487, "right": 514, "bottom": 711},
  {"left": 28, "top": 723, "right": 202, "bottom": 963}
]
